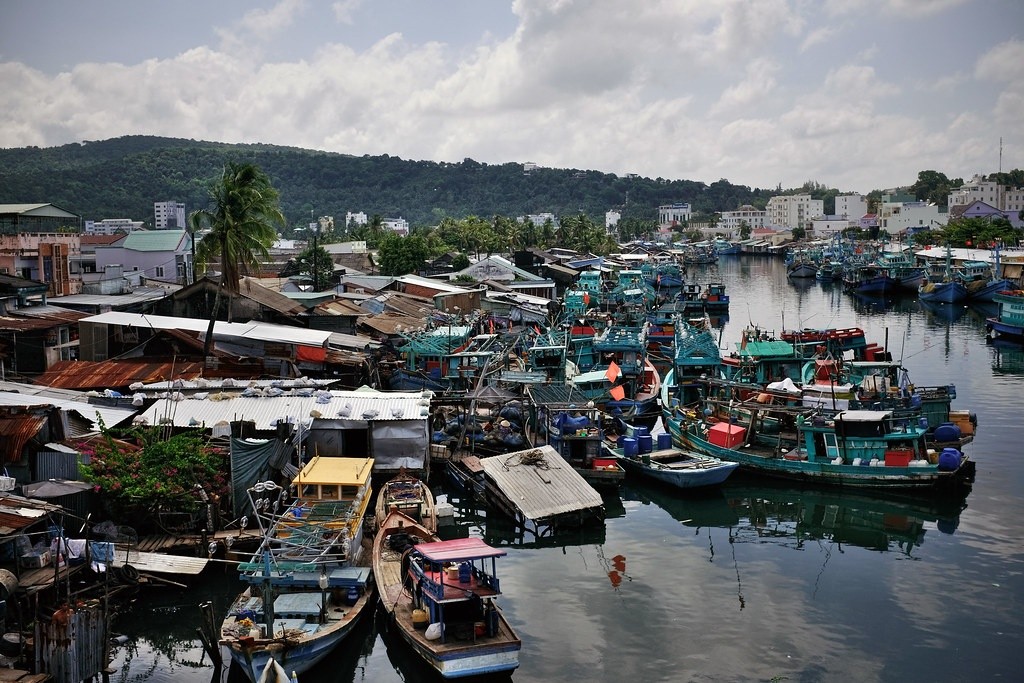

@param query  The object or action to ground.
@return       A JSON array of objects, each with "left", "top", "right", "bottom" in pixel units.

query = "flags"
[
  {"left": 856, "top": 248, "right": 862, "bottom": 254},
  {"left": 605, "top": 361, "right": 620, "bottom": 383},
  {"left": 584, "top": 292, "right": 589, "bottom": 304},
  {"left": 926, "top": 245, "right": 931, "bottom": 250},
  {"left": 610, "top": 385, "right": 625, "bottom": 401},
  {"left": 966, "top": 240, "right": 972, "bottom": 246},
  {"left": 991, "top": 241, "right": 996, "bottom": 247}
]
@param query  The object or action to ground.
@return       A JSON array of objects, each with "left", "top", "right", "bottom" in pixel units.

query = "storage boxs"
[
  {"left": 885, "top": 446, "right": 914, "bottom": 467},
  {"left": 591, "top": 454, "right": 618, "bottom": 471},
  {"left": 949, "top": 409, "right": 970, "bottom": 425},
  {"left": 708, "top": 422, "right": 747, "bottom": 450}
]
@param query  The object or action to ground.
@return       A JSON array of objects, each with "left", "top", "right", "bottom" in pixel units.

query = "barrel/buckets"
[
  {"left": 458, "top": 562, "right": 471, "bottom": 583},
  {"left": 113, "top": 636, "right": 128, "bottom": 645},
  {"left": 632, "top": 425, "right": 647, "bottom": 439},
  {"left": 448, "top": 567, "right": 458, "bottom": 580},
  {"left": 637, "top": 434, "right": 653, "bottom": 454},
  {"left": 616, "top": 435, "right": 627, "bottom": 448},
  {"left": 348, "top": 587, "right": 358, "bottom": 604},
  {"left": 474, "top": 622, "right": 487, "bottom": 637},
  {"left": 612, "top": 416, "right": 627, "bottom": 434},
  {"left": 550, "top": 409, "right": 588, "bottom": 435},
  {"left": 413, "top": 609, "right": 426, "bottom": 629},
  {"left": 0, "top": 633, "right": 26, "bottom": 659},
  {"left": 658, "top": 433, "right": 672, "bottom": 450},
  {"left": 643, "top": 454, "right": 651, "bottom": 466},
  {"left": 830, "top": 382, "right": 963, "bottom": 470},
  {"left": 623, "top": 437, "right": 638, "bottom": 457},
  {"left": 250, "top": 629, "right": 260, "bottom": 640}
]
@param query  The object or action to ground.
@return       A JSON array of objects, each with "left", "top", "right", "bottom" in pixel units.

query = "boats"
[
  {"left": 370, "top": 502, "right": 522, "bottom": 680},
  {"left": 376, "top": 463, "right": 437, "bottom": 539},
  {"left": 401, "top": 235, "right": 1023, "bottom": 512},
  {"left": 219, "top": 453, "right": 378, "bottom": 683},
  {"left": 641, "top": 479, "right": 971, "bottom": 565}
]
